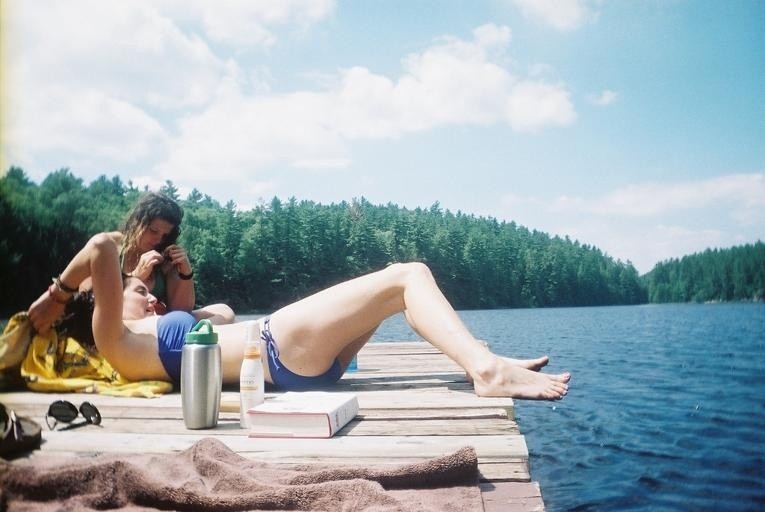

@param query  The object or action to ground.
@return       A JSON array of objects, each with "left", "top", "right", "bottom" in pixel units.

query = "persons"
[
  {"left": 77, "top": 191, "right": 196, "bottom": 316},
  {"left": 24, "top": 231, "right": 573, "bottom": 402}
]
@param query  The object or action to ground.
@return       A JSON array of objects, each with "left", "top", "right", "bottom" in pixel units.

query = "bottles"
[
  {"left": 181, "top": 319, "right": 222, "bottom": 430},
  {"left": 239, "top": 321, "right": 265, "bottom": 428}
]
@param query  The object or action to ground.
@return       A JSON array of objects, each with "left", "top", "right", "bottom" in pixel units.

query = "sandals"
[{"left": 0, "top": 399, "right": 43, "bottom": 462}]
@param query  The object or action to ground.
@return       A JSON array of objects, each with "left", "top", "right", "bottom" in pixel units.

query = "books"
[{"left": 247, "top": 389, "right": 361, "bottom": 439}]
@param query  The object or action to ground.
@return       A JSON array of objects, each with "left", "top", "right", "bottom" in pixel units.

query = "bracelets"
[
  {"left": 176, "top": 270, "right": 193, "bottom": 282},
  {"left": 47, "top": 276, "right": 80, "bottom": 304}
]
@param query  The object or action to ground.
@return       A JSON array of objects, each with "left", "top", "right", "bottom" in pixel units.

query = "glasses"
[{"left": 44, "top": 399, "right": 103, "bottom": 434}]
